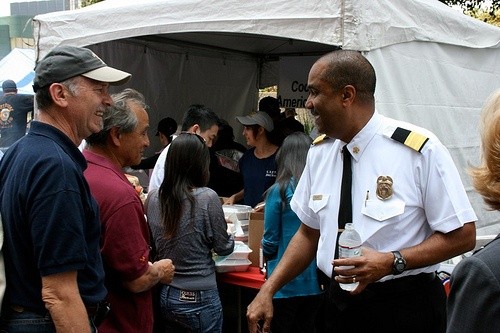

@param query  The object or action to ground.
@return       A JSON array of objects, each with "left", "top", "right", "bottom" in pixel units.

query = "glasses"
[{"left": 179, "top": 130, "right": 206, "bottom": 148}]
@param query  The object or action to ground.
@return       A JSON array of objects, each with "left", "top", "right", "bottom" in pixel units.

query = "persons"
[
  {"left": 0, "top": 43, "right": 135, "bottom": 333},
  {"left": 262, "top": 132, "right": 333, "bottom": 333},
  {"left": 144, "top": 132, "right": 235, "bottom": 333},
  {"left": 0, "top": 79, "right": 34, "bottom": 155},
  {"left": 145, "top": 104, "right": 221, "bottom": 201},
  {"left": 247, "top": 50, "right": 478, "bottom": 333},
  {"left": 446, "top": 86, "right": 500, "bottom": 333},
  {"left": 223, "top": 111, "right": 284, "bottom": 217},
  {"left": 81, "top": 89, "right": 176, "bottom": 332},
  {"left": 154, "top": 116, "right": 178, "bottom": 157}
]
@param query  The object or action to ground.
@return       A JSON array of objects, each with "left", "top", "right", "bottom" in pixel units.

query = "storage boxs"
[{"left": 248, "top": 212, "right": 265, "bottom": 268}]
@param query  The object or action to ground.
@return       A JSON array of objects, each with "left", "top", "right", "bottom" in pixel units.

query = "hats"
[
  {"left": 2, "top": 80, "right": 17, "bottom": 88},
  {"left": 235, "top": 112, "right": 274, "bottom": 131},
  {"left": 32, "top": 46, "right": 132, "bottom": 92}
]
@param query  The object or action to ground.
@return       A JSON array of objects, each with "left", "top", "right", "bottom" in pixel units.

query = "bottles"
[{"left": 338, "top": 223, "right": 362, "bottom": 292}]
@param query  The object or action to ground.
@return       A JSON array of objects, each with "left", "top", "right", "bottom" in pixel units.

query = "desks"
[{"left": 215, "top": 266, "right": 267, "bottom": 333}]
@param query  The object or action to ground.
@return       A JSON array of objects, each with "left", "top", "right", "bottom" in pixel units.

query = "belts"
[
  {"left": 82, "top": 298, "right": 111, "bottom": 316},
  {"left": 316, "top": 267, "right": 437, "bottom": 296}
]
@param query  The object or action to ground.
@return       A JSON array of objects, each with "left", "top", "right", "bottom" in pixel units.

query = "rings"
[{"left": 352, "top": 277, "right": 355, "bottom": 284}]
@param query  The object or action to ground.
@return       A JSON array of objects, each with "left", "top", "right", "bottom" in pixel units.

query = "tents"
[{"left": 23, "top": 0, "right": 500, "bottom": 277}]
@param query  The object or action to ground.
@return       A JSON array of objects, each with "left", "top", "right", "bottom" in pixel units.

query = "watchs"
[{"left": 391, "top": 250, "right": 406, "bottom": 277}]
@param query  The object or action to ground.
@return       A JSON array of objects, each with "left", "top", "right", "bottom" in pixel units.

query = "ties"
[{"left": 331, "top": 144, "right": 353, "bottom": 279}]
